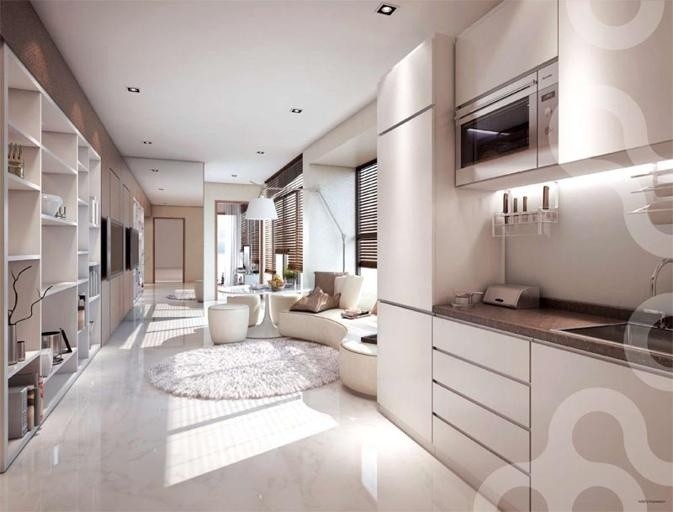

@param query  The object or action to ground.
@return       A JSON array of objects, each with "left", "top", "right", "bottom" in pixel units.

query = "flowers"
[{"left": 267, "top": 275, "right": 286, "bottom": 288}]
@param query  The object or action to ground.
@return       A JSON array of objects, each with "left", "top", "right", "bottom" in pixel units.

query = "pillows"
[{"left": 289, "top": 274, "right": 378, "bottom": 316}]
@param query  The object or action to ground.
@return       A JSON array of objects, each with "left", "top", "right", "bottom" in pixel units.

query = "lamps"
[{"left": 245, "top": 185, "right": 346, "bottom": 273}]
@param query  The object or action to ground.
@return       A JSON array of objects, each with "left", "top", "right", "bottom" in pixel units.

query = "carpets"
[{"left": 146, "top": 339, "right": 339, "bottom": 399}]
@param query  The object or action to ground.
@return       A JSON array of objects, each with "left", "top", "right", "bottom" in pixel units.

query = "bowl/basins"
[{"left": 41, "top": 193, "right": 64, "bottom": 218}]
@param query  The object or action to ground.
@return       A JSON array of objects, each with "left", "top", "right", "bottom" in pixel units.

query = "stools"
[
  {"left": 269, "top": 292, "right": 303, "bottom": 328},
  {"left": 208, "top": 296, "right": 261, "bottom": 344}
]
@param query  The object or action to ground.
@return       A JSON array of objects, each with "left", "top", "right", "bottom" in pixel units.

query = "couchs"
[{"left": 278, "top": 271, "right": 377, "bottom": 397}]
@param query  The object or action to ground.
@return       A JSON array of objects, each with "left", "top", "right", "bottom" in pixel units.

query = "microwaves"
[{"left": 454, "top": 60, "right": 558, "bottom": 191}]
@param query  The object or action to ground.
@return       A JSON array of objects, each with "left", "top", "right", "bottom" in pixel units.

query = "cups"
[
  {"left": 456, "top": 294, "right": 471, "bottom": 303},
  {"left": 15, "top": 340, "right": 26, "bottom": 361}
]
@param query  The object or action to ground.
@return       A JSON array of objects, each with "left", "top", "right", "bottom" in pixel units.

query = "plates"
[{"left": 449, "top": 300, "right": 472, "bottom": 309}]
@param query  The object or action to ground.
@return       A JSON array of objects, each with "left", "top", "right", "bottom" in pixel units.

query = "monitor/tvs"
[
  {"left": 129, "top": 228, "right": 139, "bottom": 271},
  {"left": 107, "top": 216, "right": 126, "bottom": 281}
]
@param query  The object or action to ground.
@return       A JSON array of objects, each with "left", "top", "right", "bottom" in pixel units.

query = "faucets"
[{"left": 649, "top": 258, "right": 673, "bottom": 297}]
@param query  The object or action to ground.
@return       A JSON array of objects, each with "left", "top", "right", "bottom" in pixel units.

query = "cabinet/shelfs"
[
  {"left": 0, "top": 42, "right": 100, "bottom": 473},
  {"left": 376, "top": 0, "right": 673, "bottom": 512}
]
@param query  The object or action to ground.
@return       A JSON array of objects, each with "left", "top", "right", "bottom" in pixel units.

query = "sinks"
[{"left": 550, "top": 322, "right": 673, "bottom": 358}]
